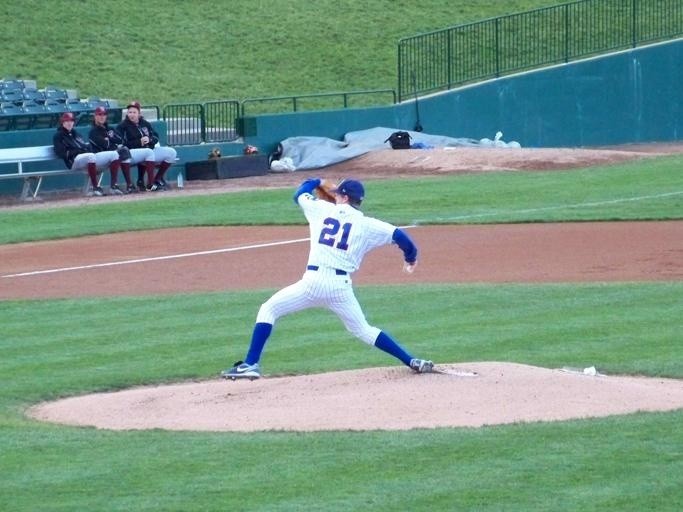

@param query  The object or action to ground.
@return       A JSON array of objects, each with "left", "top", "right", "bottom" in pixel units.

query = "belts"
[{"left": 308, "top": 265, "right": 348, "bottom": 276}]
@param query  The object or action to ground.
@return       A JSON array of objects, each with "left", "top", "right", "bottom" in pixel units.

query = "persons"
[
  {"left": 217, "top": 175, "right": 437, "bottom": 382},
  {"left": 87, "top": 105, "right": 162, "bottom": 193},
  {"left": 52, "top": 111, "right": 125, "bottom": 197},
  {"left": 112, "top": 100, "right": 179, "bottom": 191}
]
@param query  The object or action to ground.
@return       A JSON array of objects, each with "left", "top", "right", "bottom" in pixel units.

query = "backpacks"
[{"left": 385, "top": 131, "right": 411, "bottom": 149}]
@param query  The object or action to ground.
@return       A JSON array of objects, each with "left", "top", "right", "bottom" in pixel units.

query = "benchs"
[
  {"left": 0, "top": 142, "right": 179, "bottom": 205},
  {"left": 0, "top": 79, "right": 110, "bottom": 131}
]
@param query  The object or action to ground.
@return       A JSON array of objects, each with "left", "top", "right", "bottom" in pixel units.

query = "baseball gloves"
[{"left": 317, "top": 183, "right": 337, "bottom": 202}]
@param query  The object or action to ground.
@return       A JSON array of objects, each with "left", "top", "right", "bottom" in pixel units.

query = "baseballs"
[{"left": 404, "top": 265, "right": 412, "bottom": 272}]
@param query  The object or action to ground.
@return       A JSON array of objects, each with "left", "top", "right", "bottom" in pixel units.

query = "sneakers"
[
  {"left": 93, "top": 183, "right": 139, "bottom": 196},
  {"left": 412, "top": 359, "right": 434, "bottom": 374},
  {"left": 136, "top": 179, "right": 168, "bottom": 192},
  {"left": 221, "top": 361, "right": 261, "bottom": 381}
]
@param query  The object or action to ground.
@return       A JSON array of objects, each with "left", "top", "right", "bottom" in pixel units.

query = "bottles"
[{"left": 176, "top": 172, "right": 184, "bottom": 188}]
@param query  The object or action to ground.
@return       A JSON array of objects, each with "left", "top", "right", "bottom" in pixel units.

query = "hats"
[
  {"left": 95, "top": 107, "right": 107, "bottom": 115},
  {"left": 127, "top": 101, "right": 140, "bottom": 110},
  {"left": 60, "top": 112, "right": 76, "bottom": 122},
  {"left": 329, "top": 178, "right": 365, "bottom": 201}
]
